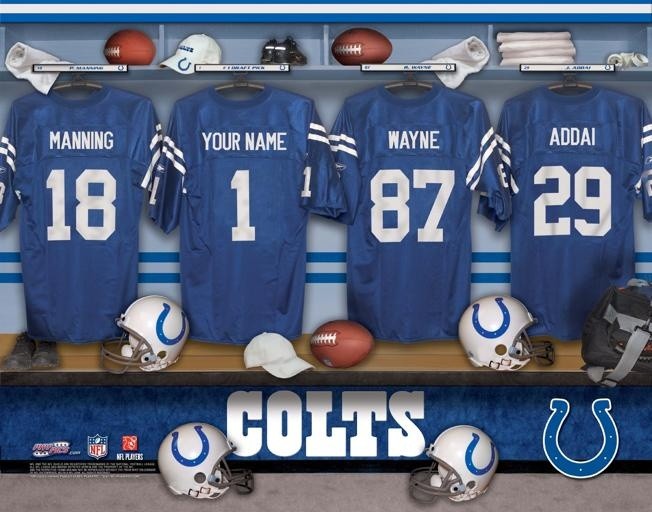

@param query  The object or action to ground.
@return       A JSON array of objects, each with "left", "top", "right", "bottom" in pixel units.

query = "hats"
[
  {"left": 243, "top": 332, "right": 314, "bottom": 379},
  {"left": 159, "top": 33, "right": 221, "bottom": 75}
]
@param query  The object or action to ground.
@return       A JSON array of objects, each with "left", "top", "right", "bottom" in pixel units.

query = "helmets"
[
  {"left": 100, "top": 295, "right": 189, "bottom": 374},
  {"left": 409, "top": 425, "right": 499, "bottom": 503},
  {"left": 459, "top": 295, "right": 554, "bottom": 369},
  {"left": 158, "top": 423, "right": 254, "bottom": 499}
]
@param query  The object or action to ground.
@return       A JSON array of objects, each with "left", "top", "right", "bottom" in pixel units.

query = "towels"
[
  {"left": 497, "top": 31, "right": 577, "bottom": 65},
  {"left": 5, "top": 39, "right": 73, "bottom": 96},
  {"left": 419, "top": 35, "right": 491, "bottom": 91}
]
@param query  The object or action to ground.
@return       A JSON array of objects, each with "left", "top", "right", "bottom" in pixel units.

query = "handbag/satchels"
[{"left": 582, "top": 286, "right": 652, "bottom": 372}]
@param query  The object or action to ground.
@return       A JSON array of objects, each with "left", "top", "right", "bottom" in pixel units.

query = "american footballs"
[
  {"left": 105, "top": 30, "right": 156, "bottom": 65},
  {"left": 331, "top": 28, "right": 392, "bottom": 65},
  {"left": 310, "top": 320, "right": 375, "bottom": 367}
]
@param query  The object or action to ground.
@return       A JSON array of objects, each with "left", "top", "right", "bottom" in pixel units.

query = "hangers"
[{"left": 11, "top": 71, "right": 643, "bottom": 114}]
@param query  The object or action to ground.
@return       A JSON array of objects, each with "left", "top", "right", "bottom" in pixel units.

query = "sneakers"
[
  {"left": 31, "top": 342, "right": 58, "bottom": 369},
  {"left": 262, "top": 39, "right": 306, "bottom": 65},
  {"left": 6, "top": 333, "right": 36, "bottom": 369}
]
